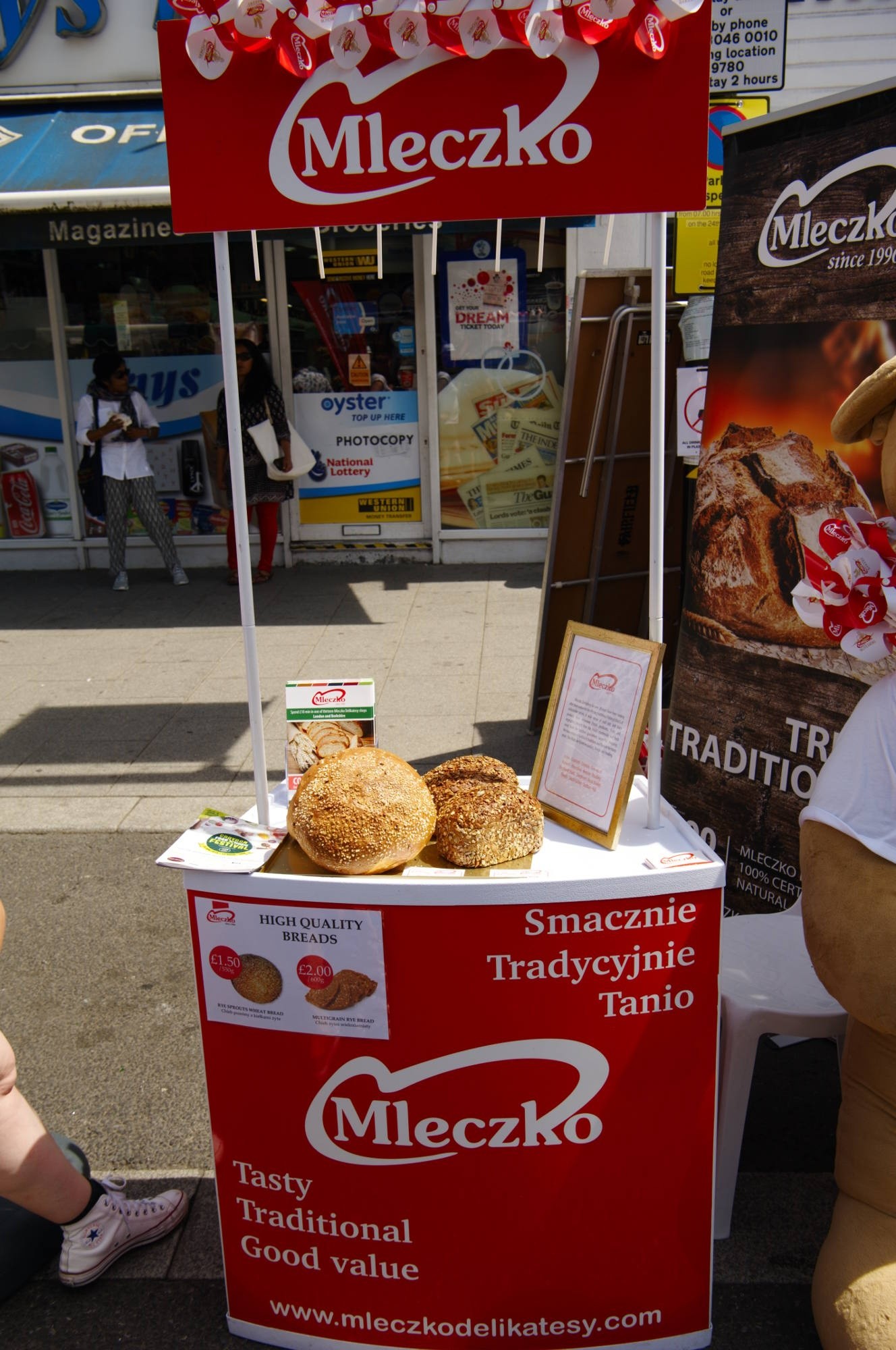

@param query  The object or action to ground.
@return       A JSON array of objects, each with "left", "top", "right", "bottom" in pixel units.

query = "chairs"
[{"left": 717, "top": 893, "right": 850, "bottom": 1236}]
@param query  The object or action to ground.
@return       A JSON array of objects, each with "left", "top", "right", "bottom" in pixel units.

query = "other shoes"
[
  {"left": 253, "top": 570, "right": 274, "bottom": 584},
  {"left": 229, "top": 570, "right": 238, "bottom": 584}
]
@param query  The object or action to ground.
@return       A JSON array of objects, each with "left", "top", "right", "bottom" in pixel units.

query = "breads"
[
  {"left": 286, "top": 747, "right": 437, "bottom": 875},
  {"left": 420, "top": 755, "right": 542, "bottom": 868},
  {"left": 301, "top": 720, "right": 364, "bottom": 758},
  {"left": 688, "top": 353, "right": 896, "bottom": 649}
]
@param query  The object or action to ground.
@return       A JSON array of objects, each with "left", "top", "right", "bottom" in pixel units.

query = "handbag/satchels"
[
  {"left": 247, "top": 419, "right": 317, "bottom": 480},
  {"left": 79, "top": 454, "right": 103, "bottom": 511}
]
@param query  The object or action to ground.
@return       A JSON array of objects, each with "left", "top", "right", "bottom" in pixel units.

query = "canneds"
[{"left": 0, "top": 469, "right": 47, "bottom": 538}]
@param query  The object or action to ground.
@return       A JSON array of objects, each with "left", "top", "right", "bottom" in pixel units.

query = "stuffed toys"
[{"left": 798, "top": 669, "right": 896, "bottom": 1350}]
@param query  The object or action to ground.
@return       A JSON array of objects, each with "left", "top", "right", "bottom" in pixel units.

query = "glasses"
[
  {"left": 113, "top": 369, "right": 130, "bottom": 379},
  {"left": 237, "top": 353, "right": 252, "bottom": 361}
]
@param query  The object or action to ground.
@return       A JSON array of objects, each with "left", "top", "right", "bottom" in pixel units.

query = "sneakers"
[
  {"left": 113, "top": 571, "right": 132, "bottom": 590},
  {"left": 171, "top": 565, "right": 188, "bottom": 585},
  {"left": 58, "top": 1168, "right": 188, "bottom": 1287}
]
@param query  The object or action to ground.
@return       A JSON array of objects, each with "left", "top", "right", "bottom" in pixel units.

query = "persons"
[
  {"left": 213, "top": 338, "right": 292, "bottom": 588},
  {"left": 75, "top": 354, "right": 192, "bottom": 591},
  {"left": 157, "top": 313, "right": 213, "bottom": 357},
  {"left": 81, "top": 281, "right": 142, "bottom": 358},
  {"left": 370, "top": 357, "right": 394, "bottom": 392},
  {"left": 293, "top": 348, "right": 337, "bottom": 393},
  {"left": 0, "top": 899, "right": 190, "bottom": 1286}
]
